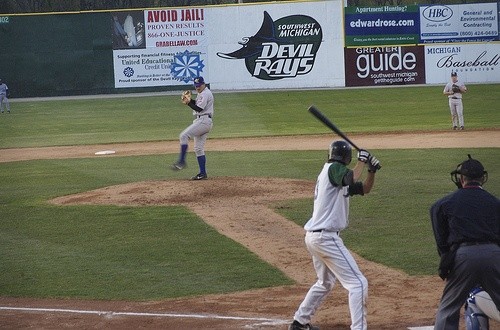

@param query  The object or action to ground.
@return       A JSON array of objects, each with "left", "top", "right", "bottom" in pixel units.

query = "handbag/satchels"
[{"left": 438, "top": 244, "right": 458, "bottom": 280}]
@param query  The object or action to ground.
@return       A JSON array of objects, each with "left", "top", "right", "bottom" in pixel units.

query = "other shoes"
[
  {"left": 7, "top": 111, "right": 10, "bottom": 115},
  {"left": 454, "top": 126, "right": 457, "bottom": 132},
  {"left": 460, "top": 125, "right": 465, "bottom": 131}
]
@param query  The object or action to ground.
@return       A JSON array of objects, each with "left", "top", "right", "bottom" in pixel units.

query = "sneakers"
[
  {"left": 171, "top": 162, "right": 186, "bottom": 170},
  {"left": 288, "top": 320, "right": 319, "bottom": 330},
  {"left": 191, "top": 173, "right": 207, "bottom": 180}
]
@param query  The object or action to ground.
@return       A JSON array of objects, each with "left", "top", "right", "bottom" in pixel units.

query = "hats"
[
  {"left": 194, "top": 77, "right": 204, "bottom": 86},
  {"left": 460, "top": 159, "right": 485, "bottom": 178},
  {"left": 451, "top": 72, "right": 457, "bottom": 77}
]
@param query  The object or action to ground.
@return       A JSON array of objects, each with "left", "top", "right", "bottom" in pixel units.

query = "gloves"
[
  {"left": 358, "top": 149, "right": 370, "bottom": 163},
  {"left": 368, "top": 156, "right": 379, "bottom": 173}
]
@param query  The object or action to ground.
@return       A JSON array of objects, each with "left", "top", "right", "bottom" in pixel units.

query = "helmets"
[{"left": 328, "top": 140, "right": 352, "bottom": 165}]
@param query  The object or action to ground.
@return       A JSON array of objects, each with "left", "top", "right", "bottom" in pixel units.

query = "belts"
[
  {"left": 313, "top": 229, "right": 338, "bottom": 235},
  {"left": 459, "top": 240, "right": 498, "bottom": 246},
  {"left": 452, "top": 97, "right": 460, "bottom": 99},
  {"left": 198, "top": 114, "right": 212, "bottom": 119}
]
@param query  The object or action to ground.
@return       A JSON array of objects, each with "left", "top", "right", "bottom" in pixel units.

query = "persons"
[
  {"left": 290, "top": 141, "right": 380, "bottom": 330},
  {"left": 172, "top": 76, "right": 214, "bottom": 180},
  {"left": 0, "top": 79, "right": 10, "bottom": 113},
  {"left": 443, "top": 72, "right": 467, "bottom": 130},
  {"left": 429, "top": 159, "right": 500, "bottom": 330}
]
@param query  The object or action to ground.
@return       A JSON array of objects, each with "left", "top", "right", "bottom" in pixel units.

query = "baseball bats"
[{"left": 306, "top": 105, "right": 382, "bottom": 171}]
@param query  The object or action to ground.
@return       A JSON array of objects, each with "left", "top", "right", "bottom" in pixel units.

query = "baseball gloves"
[
  {"left": 452, "top": 84, "right": 461, "bottom": 93},
  {"left": 180, "top": 89, "right": 192, "bottom": 104}
]
